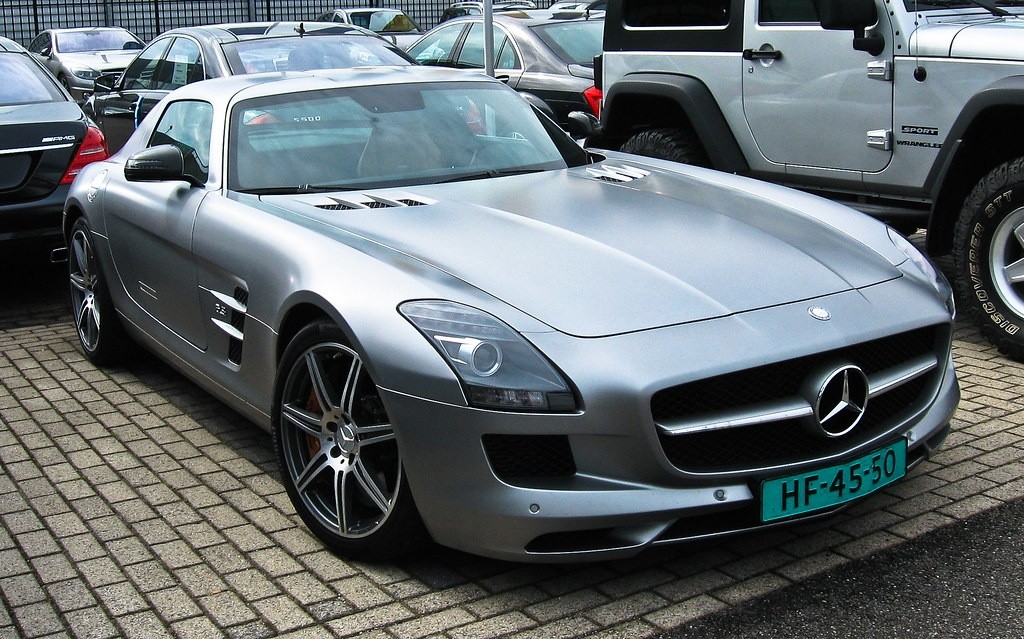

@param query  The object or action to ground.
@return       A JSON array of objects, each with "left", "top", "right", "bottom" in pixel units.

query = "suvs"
[
  {"left": 591, "top": 1, "right": 1024, "bottom": 363},
  {"left": 439, "top": 0, "right": 538, "bottom": 24}
]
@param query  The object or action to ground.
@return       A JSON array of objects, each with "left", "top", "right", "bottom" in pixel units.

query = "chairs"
[
  {"left": 196, "top": 116, "right": 302, "bottom": 189},
  {"left": 288, "top": 48, "right": 325, "bottom": 70},
  {"left": 355, "top": 112, "right": 441, "bottom": 182}
]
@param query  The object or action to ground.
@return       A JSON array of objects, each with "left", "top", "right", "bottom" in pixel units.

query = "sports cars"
[{"left": 62, "top": 61, "right": 963, "bottom": 560}]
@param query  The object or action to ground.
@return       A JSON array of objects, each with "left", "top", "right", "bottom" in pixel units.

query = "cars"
[
  {"left": 81, "top": 20, "right": 486, "bottom": 155},
  {"left": 29, "top": 25, "right": 150, "bottom": 116},
  {"left": 0, "top": 37, "right": 111, "bottom": 274},
  {"left": 549, "top": 0, "right": 607, "bottom": 10},
  {"left": 405, "top": 11, "right": 606, "bottom": 145},
  {"left": 317, "top": 8, "right": 427, "bottom": 52}
]
